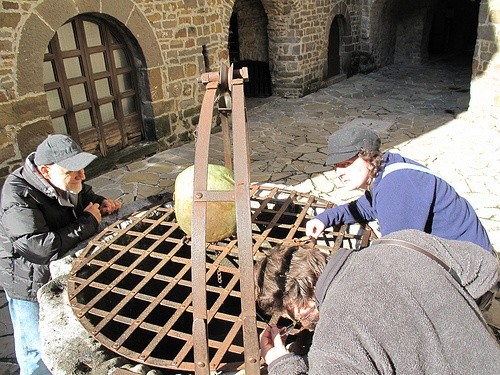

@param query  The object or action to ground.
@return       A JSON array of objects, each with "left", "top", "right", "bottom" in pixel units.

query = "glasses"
[{"left": 257, "top": 313, "right": 297, "bottom": 337}]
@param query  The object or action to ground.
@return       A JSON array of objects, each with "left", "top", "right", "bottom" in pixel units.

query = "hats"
[
  {"left": 33, "top": 133, "right": 100, "bottom": 172},
  {"left": 323, "top": 126, "right": 382, "bottom": 164}
]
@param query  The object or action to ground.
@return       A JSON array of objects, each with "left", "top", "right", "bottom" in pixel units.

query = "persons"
[
  {"left": 1, "top": 133, "right": 123, "bottom": 375},
  {"left": 305, "top": 126, "right": 495, "bottom": 253},
  {"left": 253, "top": 227, "right": 500, "bottom": 375}
]
